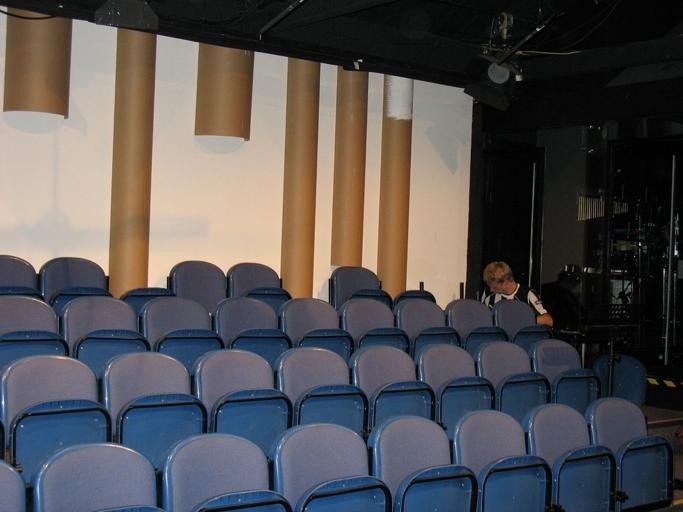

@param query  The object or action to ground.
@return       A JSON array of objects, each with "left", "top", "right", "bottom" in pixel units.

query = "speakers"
[{"left": 463, "top": 48, "right": 522, "bottom": 113}]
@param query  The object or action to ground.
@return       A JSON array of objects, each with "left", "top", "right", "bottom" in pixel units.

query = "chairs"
[{"left": 0, "top": 255, "right": 674, "bottom": 510}]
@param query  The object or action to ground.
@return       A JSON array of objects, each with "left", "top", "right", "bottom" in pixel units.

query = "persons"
[
  {"left": 481, "top": 260, "right": 556, "bottom": 328},
  {"left": 538, "top": 264, "right": 590, "bottom": 336}
]
